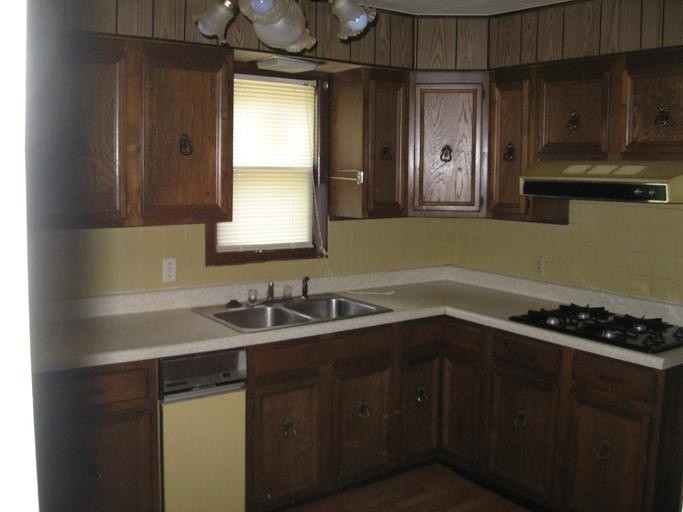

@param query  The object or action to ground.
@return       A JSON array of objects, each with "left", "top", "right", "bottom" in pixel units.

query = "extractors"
[{"left": 516, "top": 159, "right": 682, "bottom": 206}]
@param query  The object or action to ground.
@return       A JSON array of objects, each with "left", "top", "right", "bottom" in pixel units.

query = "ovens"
[{"left": 157, "top": 381, "right": 247, "bottom": 511}]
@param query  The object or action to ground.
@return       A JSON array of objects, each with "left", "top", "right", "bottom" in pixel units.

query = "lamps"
[{"left": 190, "top": 0, "right": 379, "bottom": 55}]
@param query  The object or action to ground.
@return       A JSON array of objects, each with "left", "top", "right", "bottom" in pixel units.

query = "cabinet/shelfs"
[
  {"left": 538, "top": 46, "right": 683, "bottom": 161},
  {"left": 29, "top": 359, "right": 158, "bottom": 511},
  {"left": 329, "top": 66, "right": 409, "bottom": 221},
  {"left": 483, "top": 327, "right": 565, "bottom": 512},
  {"left": 407, "top": 71, "right": 491, "bottom": 220},
  {"left": 247, "top": 314, "right": 439, "bottom": 510},
  {"left": 559, "top": 350, "right": 683, "bottom": 511},
  {"left": 440, "top": 316, "right": 486, "bottom": 490},
  {"left": 491, "top": 65, "right": 569, "bottom": 227},
  {"left": 26, "top": 25, "right": 234, "bottom": 230}
]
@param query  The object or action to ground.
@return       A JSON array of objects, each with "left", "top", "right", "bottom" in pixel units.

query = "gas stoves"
[{"left": 508, "top": 302, "right": 682, "bottom": 355}]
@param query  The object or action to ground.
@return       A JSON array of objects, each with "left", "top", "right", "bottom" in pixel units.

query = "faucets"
[
  {"left": 266, "top": 281, "right": 274, "bottom": 303},
  {"left": 301, "top": 276, "right": 309, "bottom": 300}
]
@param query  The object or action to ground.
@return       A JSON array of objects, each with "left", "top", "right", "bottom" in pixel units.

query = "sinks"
[
  {"left": 282, "top": 293, "right": 376, "bottom": 321},
  {"left": 213, "top": 305, "right": 312, "bottom": 330}
]
